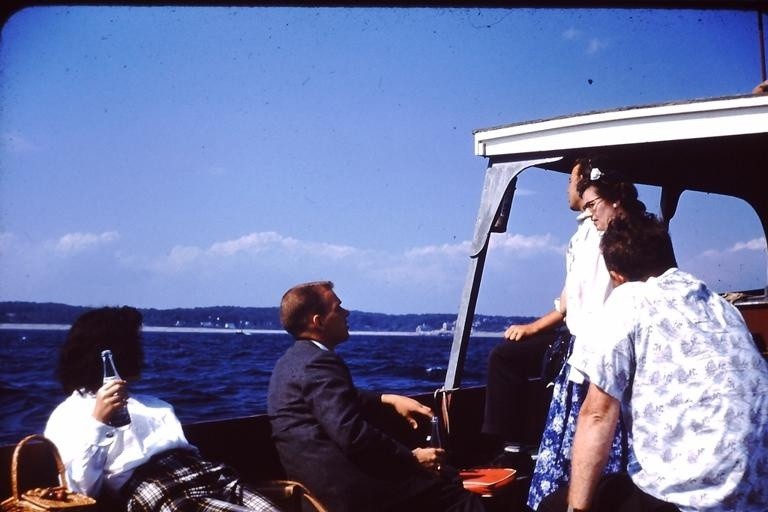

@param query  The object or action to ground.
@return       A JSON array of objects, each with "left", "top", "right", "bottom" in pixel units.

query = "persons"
[
  {"left": 41, "top": 303, "right": 284, "bottom": 511},
  {"left": 266, "top": 278, "right": 489, "bottom": 510},
  {"left": 501, "top": 150, "right": 602, "bottom": 341},
  {"left": 537, "top": 208, "right": 767, "bottom": 511},
  {"left": 526, "top": 171, "right": 646, "bottom": 510}
]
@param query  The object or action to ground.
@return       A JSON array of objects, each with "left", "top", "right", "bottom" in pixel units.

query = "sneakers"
[{"left": 488, "top": 447, "right": 533, "bottom": 473}]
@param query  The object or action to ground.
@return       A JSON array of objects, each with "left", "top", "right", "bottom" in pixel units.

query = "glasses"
[{"left": 583, "top": 196, "right": 604, "bottom": 212}]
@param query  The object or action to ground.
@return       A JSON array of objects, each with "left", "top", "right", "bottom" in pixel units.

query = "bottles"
[
  {"left": 428, "top": 415, "right": 439, "bottom": 449},
  {"left": 100, "top": 351, "right": 130, "bottom": 429}
]
